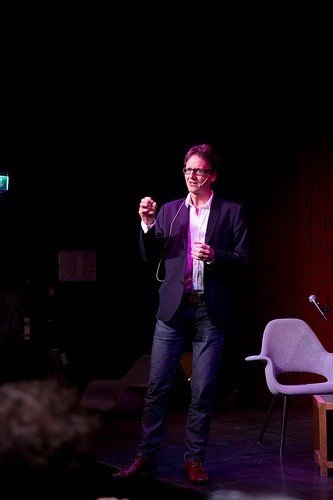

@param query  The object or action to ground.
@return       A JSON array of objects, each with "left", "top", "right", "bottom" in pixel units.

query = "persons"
[
  {"left": 0, "top": 381, "right": 100, "bottom": 500},
  {"left": 113, "top": 143, "right": 256, "bottom": 484}
]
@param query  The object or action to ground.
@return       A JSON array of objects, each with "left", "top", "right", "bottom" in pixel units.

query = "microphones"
[
  {"left": 308, "top": 294, "right": 329, "bottom": 321},
  {"left": 198, "top": 172, "right": 211, "bottom": 187}
]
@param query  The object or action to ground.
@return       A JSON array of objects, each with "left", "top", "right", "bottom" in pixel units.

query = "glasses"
[{"left": 183, "top": 168, "right": 213, "bottom": 176}]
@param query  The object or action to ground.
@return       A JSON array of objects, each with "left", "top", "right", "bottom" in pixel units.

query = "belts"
[{"left": 182, "top": 294, "right": 204, "bottom": 302}]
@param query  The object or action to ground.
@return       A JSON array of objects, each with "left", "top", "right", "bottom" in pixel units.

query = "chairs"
[
  {"left": 245, "top": 318, "right": 333, "bottom": 457},
  {"left": 79, "top": 355, "right": 152, "bottom": 412}
]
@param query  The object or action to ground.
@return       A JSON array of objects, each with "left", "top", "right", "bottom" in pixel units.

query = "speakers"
[
  {"left": 80, "top": 380, "right": 125, "bottom": 410},
  {"left": 122, "top": 355, "right": 152, "bottom": 387}
]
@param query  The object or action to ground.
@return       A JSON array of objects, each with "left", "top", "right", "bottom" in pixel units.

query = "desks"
[{"left": 312, "top": 395, "right": 333, "bottom": 477}]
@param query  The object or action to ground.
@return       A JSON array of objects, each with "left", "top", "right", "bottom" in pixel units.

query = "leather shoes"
[
  {"left": 114, "top": 456, "right": 146, "bottom": 478},
  {"left": 184, "top": 460, "right": 208, "bottom": 484}
]
